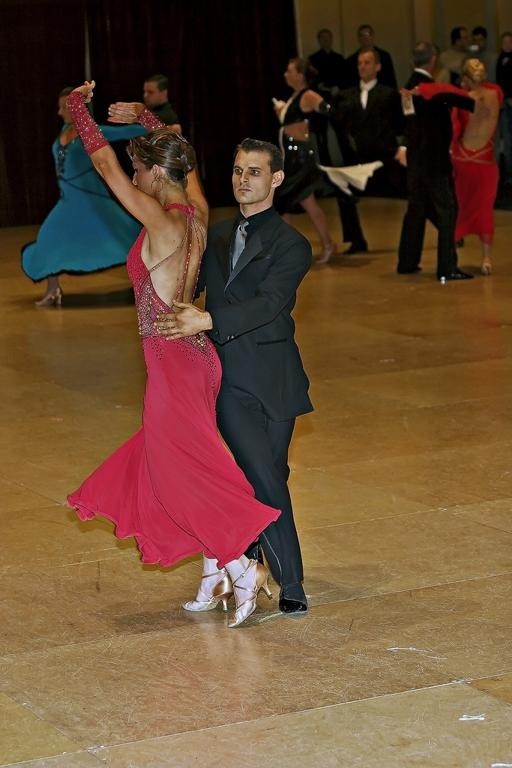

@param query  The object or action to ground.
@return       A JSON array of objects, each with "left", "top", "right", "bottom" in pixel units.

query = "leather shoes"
[
  {"left": 316, "top": 242, "right": 368, "bottom": 264},
  {"left": 437, "top": 268, "right": 473, "bottom": 280}
]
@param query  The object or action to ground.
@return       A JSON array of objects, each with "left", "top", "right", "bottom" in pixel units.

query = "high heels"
[
  {"left": 35, "top": 287, "right": 61, "bottom": 306},
  {"left": 182, "top": 557, "right": 308, "bottom": 627}
]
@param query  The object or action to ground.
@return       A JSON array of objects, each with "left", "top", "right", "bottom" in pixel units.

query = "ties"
[{"left": 232, "top": 220, "right": 249, "bottom": 270}]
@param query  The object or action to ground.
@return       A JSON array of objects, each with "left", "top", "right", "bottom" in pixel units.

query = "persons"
[
  {"left": 144, "top": 74, "right": 179, "bottom": 128},
  {"left": 67, "top": 79, "right": 282, "bottom": 627},
  {"left": 397, "top": 40, "right": 474, "bottom": 280},
  {"left": 153, "top": 137, "right": 315, "bottom": 616},
  {"left": 306, "top": 23, "right": 512, "bottom": 161},
  {"left": 332, "top": 44, "right": 400, "bottom": 256},
  {"left": 20, "top": 85, "right": 149, "bottom": 307},
  {"left": 398, "top": 58, "right": 505, "bottom": 275},
  {"left": 271, "top": 57, "right": 336, "bottom": 266}
]
